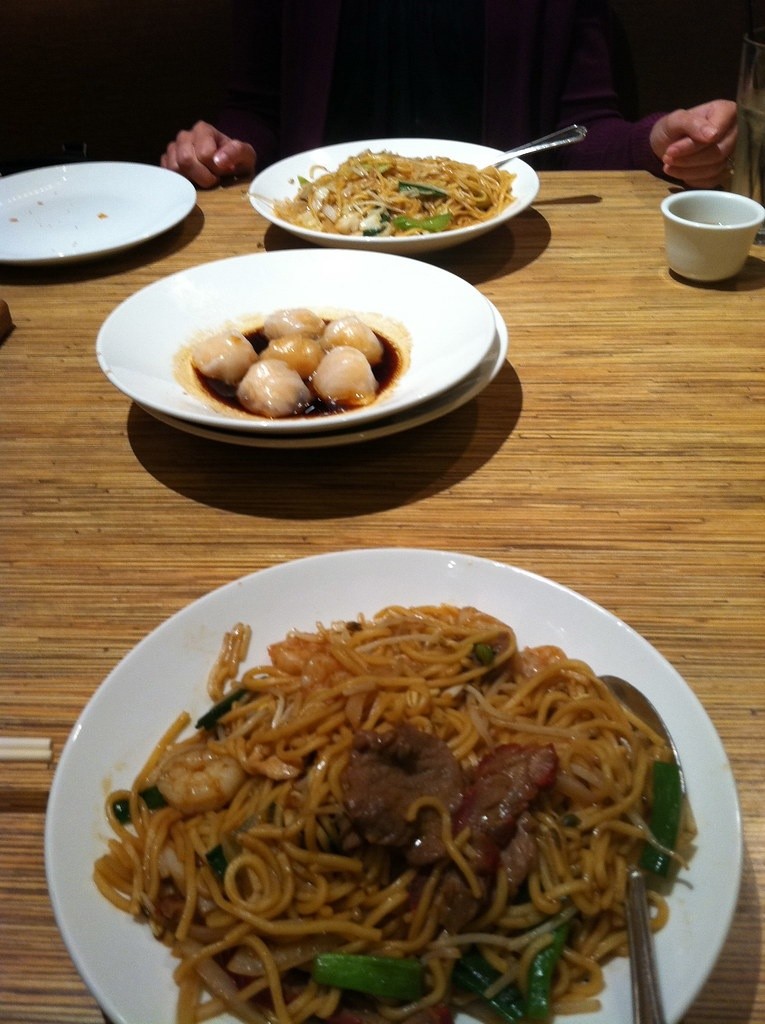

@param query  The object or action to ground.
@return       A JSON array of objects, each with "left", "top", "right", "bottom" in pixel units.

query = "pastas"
[
  {"left": 295, "top": 149, "right": 515, "bottom": 235},
  {"left": 92, "top": 605, "right": 699, "bottom": 1024}
]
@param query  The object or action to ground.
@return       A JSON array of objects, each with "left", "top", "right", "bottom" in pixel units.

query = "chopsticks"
[{"left": 0, "top": 734, "right": 53, "bottom": 767}]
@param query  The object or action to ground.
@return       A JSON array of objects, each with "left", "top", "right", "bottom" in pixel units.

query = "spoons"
[{"left": 583, "top": 673, "right": 688, "bottom": 1022}]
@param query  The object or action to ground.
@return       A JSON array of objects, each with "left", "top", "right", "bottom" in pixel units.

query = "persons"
[{"left": 130, "top": 0, "right": 741, "bottom": 192}]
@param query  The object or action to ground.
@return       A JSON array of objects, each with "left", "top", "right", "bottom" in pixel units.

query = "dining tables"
[{"left": 0, "top": 156, "right": 765, "bottom": 1024}]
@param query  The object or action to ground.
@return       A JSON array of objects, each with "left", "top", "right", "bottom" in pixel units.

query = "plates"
[
  {"left": 248, "top": 139, "right": 541, "bottom": 258},
  {"left": 95, "top": 246, "right": 498, "bottom": 435},
  {"left": 41, "top": 547, "right": 745, "bottom": 1024},
  {"left": 1, "top": 159, "right": 197, "bottom": 263},
  {"left": 133, "top": 286, "right": 508, "bottom": 450}
]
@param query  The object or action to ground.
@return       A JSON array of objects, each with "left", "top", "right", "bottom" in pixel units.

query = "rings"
[{"left": 725, "top": 153, "right": 736, "bottom": 177}]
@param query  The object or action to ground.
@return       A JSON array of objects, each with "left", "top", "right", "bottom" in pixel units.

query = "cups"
[{"left": 661, "top": 186, "right": 765, "bottom": 283}]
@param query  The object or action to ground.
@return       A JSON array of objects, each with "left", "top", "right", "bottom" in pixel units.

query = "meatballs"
[{"left": 192, "top": 307, "right": 385, "bottom": 418}]
